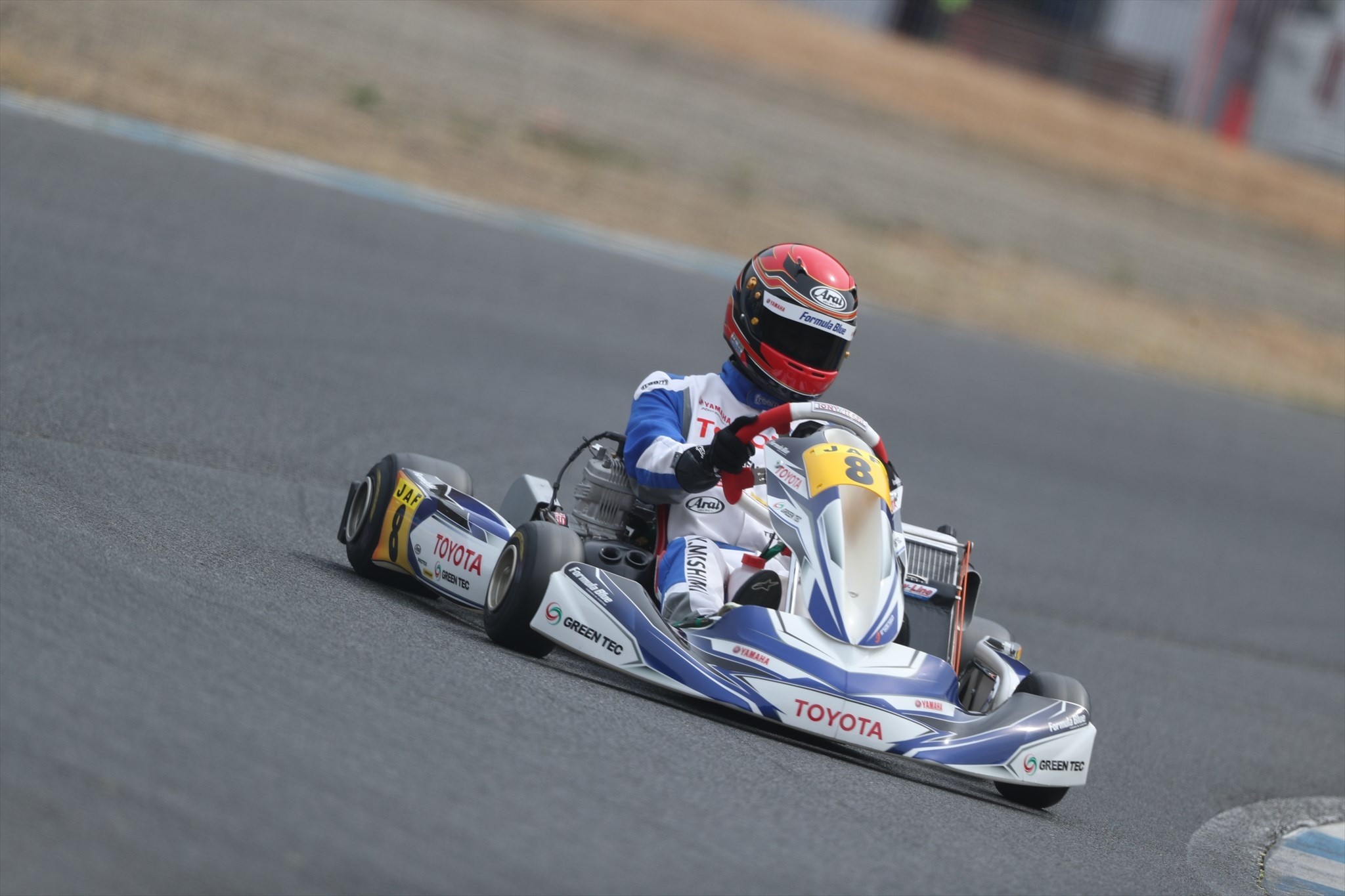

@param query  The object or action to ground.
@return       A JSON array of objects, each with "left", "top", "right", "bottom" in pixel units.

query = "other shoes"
[
  {"left": 728, "top": 568, "right": 782, "bottom": 610},
  {"left": 894, "top": 611, "right": 911, "bottom": 646}
]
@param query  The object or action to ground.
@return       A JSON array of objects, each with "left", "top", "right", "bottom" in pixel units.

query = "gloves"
[{"left": 707, "top": 415, "right": 759, "bottom": 475}]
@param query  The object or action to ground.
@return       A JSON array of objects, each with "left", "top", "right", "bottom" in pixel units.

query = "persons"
[{"left": 624, "top": 243, "right": 910, "bottom": 648}]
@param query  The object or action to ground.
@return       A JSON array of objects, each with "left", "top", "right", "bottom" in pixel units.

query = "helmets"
[{"left": 722, "top": 242, "right": 857, "bottom": 404}]
